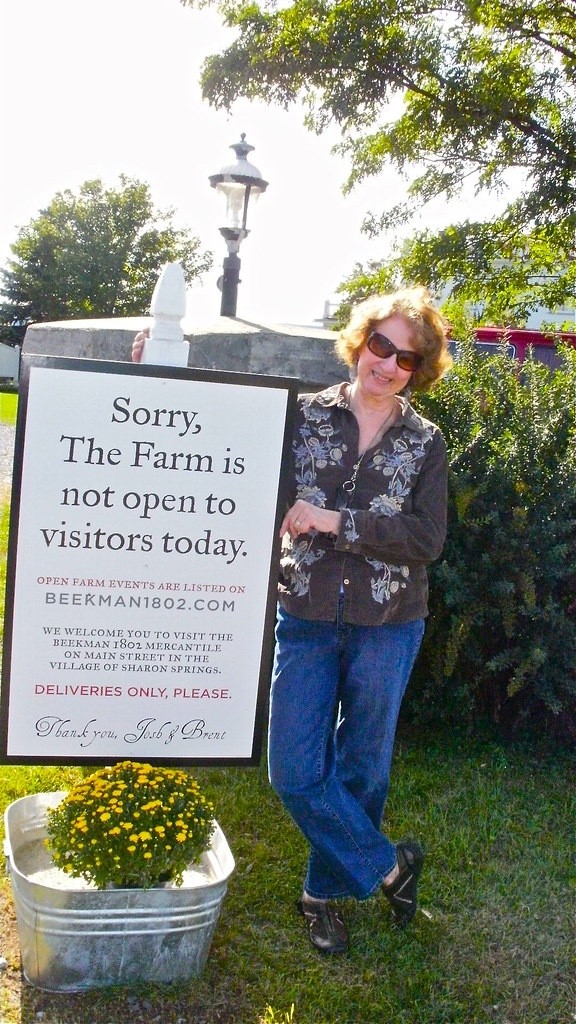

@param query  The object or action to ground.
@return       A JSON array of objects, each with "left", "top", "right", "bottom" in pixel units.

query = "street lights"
[{"left": 207, "top": 131, "right": 269, "bottom": 316}]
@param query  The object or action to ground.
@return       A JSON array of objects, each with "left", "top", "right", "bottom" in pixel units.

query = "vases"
[{"left": 4, "top": 790, "right": 236, "bottom": 994}]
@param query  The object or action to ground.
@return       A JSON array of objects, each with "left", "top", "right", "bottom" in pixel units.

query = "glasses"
[{"left": 367, "top": 328, "right": 424, "bottom": 372}]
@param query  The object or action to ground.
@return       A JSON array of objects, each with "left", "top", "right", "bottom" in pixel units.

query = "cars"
[{"left": 444, "top": 325, "right": 576, "bottom": 387}]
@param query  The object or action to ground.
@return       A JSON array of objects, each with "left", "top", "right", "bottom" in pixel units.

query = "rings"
[{"left": 295, "top": 520, "right": 300, "bottom": 525}]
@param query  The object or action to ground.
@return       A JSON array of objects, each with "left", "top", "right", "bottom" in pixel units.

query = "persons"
[{"left": 131, "top": 286, "right": 450, "bottom": 955}]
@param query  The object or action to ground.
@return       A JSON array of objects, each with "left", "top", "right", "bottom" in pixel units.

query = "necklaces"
[{"left": 342, "top": 386, "right": 395, "bottom": 492}]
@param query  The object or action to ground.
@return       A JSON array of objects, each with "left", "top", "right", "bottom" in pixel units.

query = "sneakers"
[
  {"left": 296, "top": 890, "right": 350, "bottom": 956},
  {"left": 380, "top": 843, "right": 426, "bottom": 929}
]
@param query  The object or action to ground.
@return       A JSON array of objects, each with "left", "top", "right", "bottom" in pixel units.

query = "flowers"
[{"left": 43, "top": 759, "right": 218, "bottom": 893}]
